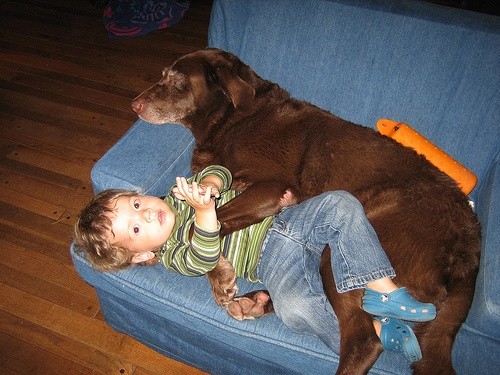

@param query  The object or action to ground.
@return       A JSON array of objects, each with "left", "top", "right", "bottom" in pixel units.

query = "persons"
[{"left": 72, "top": 163, "right": 437, "bottom": 363}]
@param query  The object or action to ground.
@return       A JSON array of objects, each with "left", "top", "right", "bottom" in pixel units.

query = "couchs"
[{"left": 69, "top": 0, "right": 500, "bottom": 375}]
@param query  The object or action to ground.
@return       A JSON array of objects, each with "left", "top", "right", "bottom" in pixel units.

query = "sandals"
[{"left": 363, "top": 285, "right": 436, "bottom": 362}]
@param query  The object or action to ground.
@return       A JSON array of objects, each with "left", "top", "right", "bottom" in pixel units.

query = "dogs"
[{"left": 131, "top": 47, "right": 483, "bottom": 375}]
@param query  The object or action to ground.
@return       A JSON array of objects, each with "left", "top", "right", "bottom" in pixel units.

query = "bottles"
[{"left": 376, "top": 118, "right": 477, "bottom": 197}]
